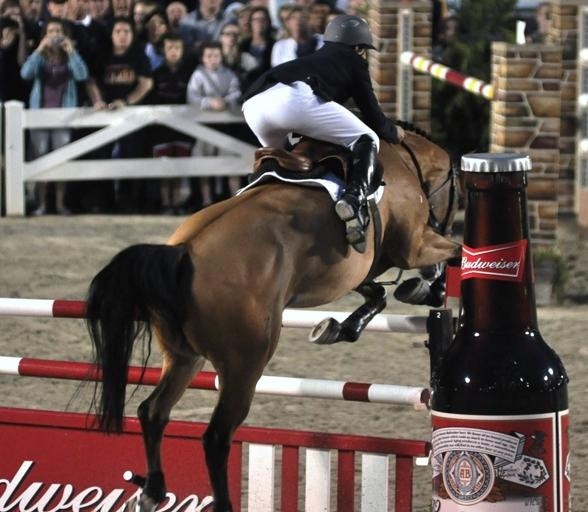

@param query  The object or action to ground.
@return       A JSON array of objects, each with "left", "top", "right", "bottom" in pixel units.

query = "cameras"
[{"left": 51, "top": 37, "right": 64, "bottom": 46}]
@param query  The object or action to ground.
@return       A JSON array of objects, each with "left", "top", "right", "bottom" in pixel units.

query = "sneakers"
[
  {"left": 55, "top": 205, "right": 74, "bottom": 216},
  {"left": 28, "top": 207, "right": 48, "bottom": 217}
]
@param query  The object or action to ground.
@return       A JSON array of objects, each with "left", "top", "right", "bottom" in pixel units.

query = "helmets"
[{"left": 321, "top": 13, "right": 377, "bottom": 52}]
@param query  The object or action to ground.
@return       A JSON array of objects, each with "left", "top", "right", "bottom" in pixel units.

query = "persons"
[
  {"left": 237, "top": 13, "right": 407, "bottom": 254},
  {"left": 1, "top": 0, "right": 349, "bottom": 207}
]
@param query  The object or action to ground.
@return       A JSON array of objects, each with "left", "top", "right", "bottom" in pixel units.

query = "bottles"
[{"left": 427, "top": 152, "right": 570, "bottom": 512}]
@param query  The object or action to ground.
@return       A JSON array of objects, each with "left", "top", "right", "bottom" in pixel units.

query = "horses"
[{"left": 60, "top": 118, "right": 468, "bottom": 512}]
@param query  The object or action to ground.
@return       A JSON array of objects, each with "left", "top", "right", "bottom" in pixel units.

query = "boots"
[{"left": 332, "top": 129, "right": 386, "bottom": 256}]
